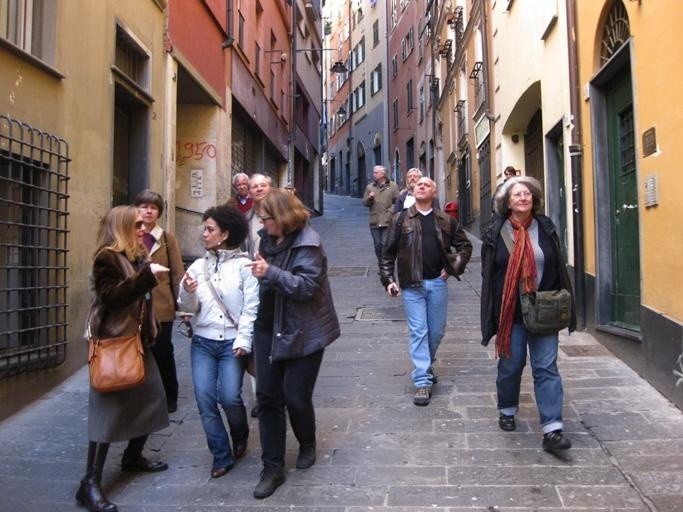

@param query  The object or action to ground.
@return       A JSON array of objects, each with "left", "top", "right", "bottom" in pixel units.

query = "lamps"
[
  {"left": 323, "top": 98, "right": 347, "bottom": 118},
  {"left": 296, "top": 46, "right": 349, "bottom": 74}
]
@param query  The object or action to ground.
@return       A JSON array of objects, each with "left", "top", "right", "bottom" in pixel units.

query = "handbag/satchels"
[
  {"left": 86, "top": 332, "right": 147, "bottom": 395},
  {"left": 518, "top": 287, "right": 573, "bottom": 335}
]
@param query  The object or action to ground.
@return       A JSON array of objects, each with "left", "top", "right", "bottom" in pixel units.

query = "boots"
[
  {"left": 120, "top": 435, "right": 169, "bottom": 472},
  {"left": 75, "top": 441, "right": 117, "bottom": 512}
]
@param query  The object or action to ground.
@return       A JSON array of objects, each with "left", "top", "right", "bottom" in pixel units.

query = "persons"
[
  {"left": 377, "top": 176, "right": 473, "bottom": 406},
  {"left": 133, "top": 188, "right": 186, "bottom": 414},
  {"left": 490, "top": 166, "right": 516, "bottom": 212},
  {"left": 74, "top": 204, "right": 171, "bottom": 511},
  {"left": 225, "top": 173, "right": 254, "bottom": 213},
  {"left": 361, "top": 165, "right": 400, "bottom": 292},
  {"left": 243, "top": 173, "right": 272, "bottom": 416},
  {"left": 480, "top": 176, "right": 577, "bottom": 451},
  {"left": 176, "top": 205, "right": 261, "bottom": 479},
  {"left": 393, "top": 167, "right": 423, "bottom": 214},
  {"left": 243, "top": 186, "right": 341, "bottom": 499}
]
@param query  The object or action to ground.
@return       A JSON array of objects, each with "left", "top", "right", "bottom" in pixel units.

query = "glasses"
[
  {"left": 134, "top": 220, "right": 144, "bottom": 230},
  {"left": 257, "top": 213, "right": 275, "bottom": 223}
]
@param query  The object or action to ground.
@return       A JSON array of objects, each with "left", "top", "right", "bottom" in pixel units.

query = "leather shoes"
[
  {"left": 209, "top": 465, "right": 230, "bottom": 478},
  {"left": 232, "top": 439, "right": 245, "bottom": 460}
]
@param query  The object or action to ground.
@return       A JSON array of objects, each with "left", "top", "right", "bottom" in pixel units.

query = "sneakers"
[
  {"left": 541, "top": 431, "right": 571, "bottom": 454},
  {"left": 412, "top": 386, "right": 432, "bottom": 406},
  {"left": 498, "top": 413, "right": 515, "bottom": 433},
  {"left": 250, "top": 465, "right": 285, "bottom": 500},
  {"left": 295, "top": 442, "right": 315, "bottom": 469},
  {"left": 428, "top": 365, "right": 437, "bottom": 385}
]
